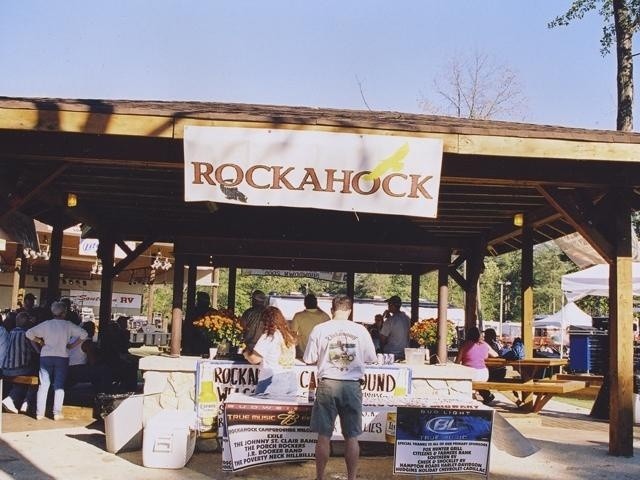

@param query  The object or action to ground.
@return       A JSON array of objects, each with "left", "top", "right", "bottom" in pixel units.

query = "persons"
[
  {"left": 242, "top": 306, "right": 298, "bottom": 397},
  {"left": 373, "top": 315, "right": 384, "bottom": 330},
  {"left": 194, "top": 291, "right": 218, "bottom": 314},
  {"left": 0, "top": 293, "right": 138, "bottom": 420},
  {"left": 242, "top": 289, "right": 267, "bottom": 326},
  {"left": 292, "top": 294, "right": 330, "bottom": 359},
  {"left": 381, "top": 295, "right": 410, "bottom": 360},
  {"left": 457, "top": 325, "right": 498, "bottom": 381},
  {"left": 478, "top": 327, "right": 505, "bottom": 403},
  {"left": 301, "top": 294, "right": 378, "bottom": 480}
]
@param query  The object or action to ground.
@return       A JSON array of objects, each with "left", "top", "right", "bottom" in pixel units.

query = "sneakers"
[
  {"left": 2, "top": 396, "right": 36, "bottom": 418},
  {"left": 36, "top": 414, "right": 65, "bottom": 421}
]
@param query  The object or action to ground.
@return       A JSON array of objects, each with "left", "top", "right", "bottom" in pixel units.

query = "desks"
[{"left": 484, "top": 356, "right": 568, "bottom": 412}]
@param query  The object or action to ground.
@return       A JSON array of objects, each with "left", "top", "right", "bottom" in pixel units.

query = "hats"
[
  {"left": 24, "top": 293, "right": 37, "bottom": 300},
  {"left": 384, "top": 295, "right": 403, "bottom": 307},
  {"left": 248, "top": 290, "right": 266, "bottom": 302}
]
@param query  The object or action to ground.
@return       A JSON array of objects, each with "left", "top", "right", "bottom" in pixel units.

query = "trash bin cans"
[
  {"left": 533, "top": 348, "right": 561, "bottom": 395},
  {"left": 631, "top": 375, "right": 640, "bottom": 427},
  {"left": 96, "top": 392, "right": 143, "bottom": 455}
]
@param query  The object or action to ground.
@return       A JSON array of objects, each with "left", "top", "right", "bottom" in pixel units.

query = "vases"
[{"left": 208, "top": 347, "right": 218, "bottom": 359}]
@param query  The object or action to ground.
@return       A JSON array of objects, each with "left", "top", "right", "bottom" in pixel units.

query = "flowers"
[
  {"left": 409, "top": 318, "right": 455, "bottom": 347},
  {"left": 193, "top": 315, "right": 247, "bottom": 350}
]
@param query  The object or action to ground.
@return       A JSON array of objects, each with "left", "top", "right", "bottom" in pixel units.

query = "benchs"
[
  {"left": 0, "top": 374, "right": 40, "bottom": 399},
  {"left": 472, "top": 379, "right": 586, "bottom": 412}
]
